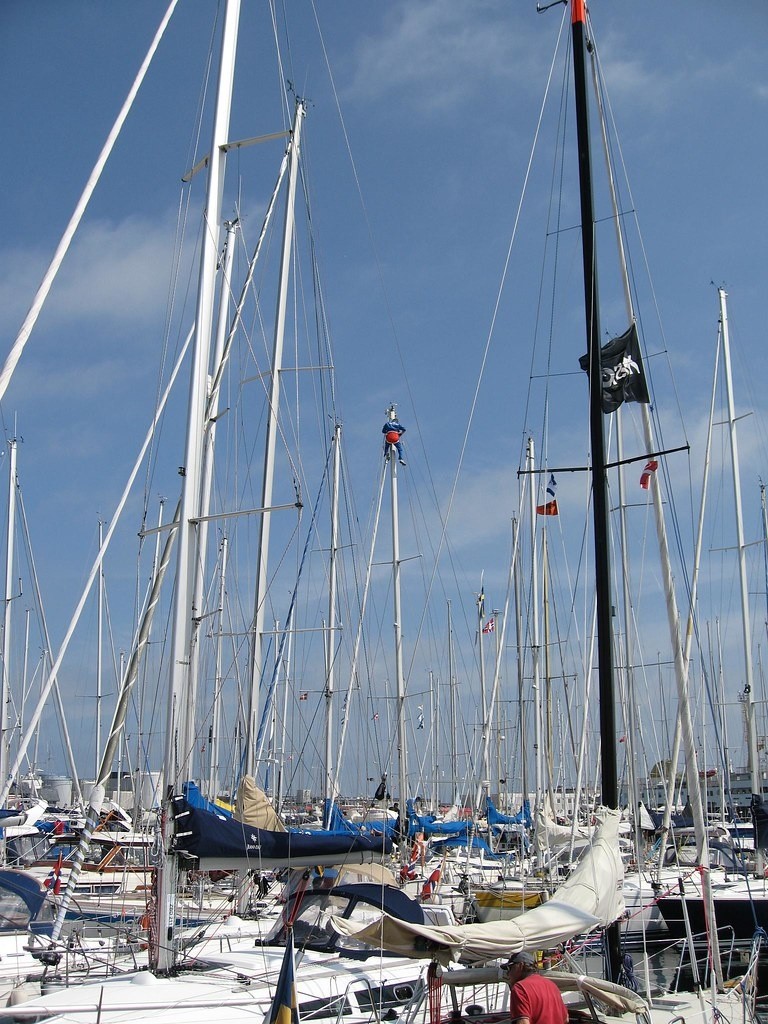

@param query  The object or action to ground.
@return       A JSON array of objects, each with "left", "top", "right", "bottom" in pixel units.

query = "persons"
[
  {"left": 389, "top": 803, "right": 400, "bottom": 821},
  {"left": 506, "top": 952, "right": 569, "bottom": 1024},
  {"left": 382, "top": 418, "right": 407, "bottom": 466},
  {"left": 750, "top": 795, "right": 768, "bottom": 878},
  {"left": 406, "top": 797, "right": 421, "bottom": 817}
]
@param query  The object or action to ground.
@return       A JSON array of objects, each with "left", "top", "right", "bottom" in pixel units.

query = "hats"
[{"left": 500, "top": 952, "right": 538, "bottom": 970}]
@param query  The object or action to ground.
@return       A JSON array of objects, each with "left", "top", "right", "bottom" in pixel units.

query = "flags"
[
  {"left": 43, "top": 852, "right": 63, "bottom": 894},
  {"left": 640, "top": 461, "right": 658, "bottom": 489},
  {"left": 340, "top": 693, "right": 346, "bottom": 725},
  {"left": 476, "top": 586, "right": 486, "bottom": 619},
  {"left": 200, "top": 743, "right": 206, "bottom": 752},
  {"left": 482, "top": 617, "right": 495, "bottom": 634},
  {"left": 536, "top": 500, "right": 559, "bottom": 516},
  {"left": 287, "top": 754, "right": 292, "bottom": 760},
  {"left": 371, "top": 713, "right": 379, "bottom": 720},
  {"left": 400, "top": 840, "right": 422, "bottom": 887},
  {"left": 420, "top": 860, "right": 443, "bottom": 900},
  {"left": 416, "top": 704, "right": 424, "bottom": 730},
  {"left": 620, "top": 736, "right": 625, "bottom": 744},
  {"left": 299, "top": 691, "right": 308, "bottom": 701},
  {"left": 547, "top": 474, "right": 557, "bottom": 499}
]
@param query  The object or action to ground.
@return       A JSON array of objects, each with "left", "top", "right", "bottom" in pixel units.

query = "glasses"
[{"left": 507, "top": 963, "right": 519, "bottom": 971}]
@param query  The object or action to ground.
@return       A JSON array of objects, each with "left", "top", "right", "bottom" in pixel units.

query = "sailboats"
[{"left": 0, "top": 1, "right": 766, "bottom": 1024}]
